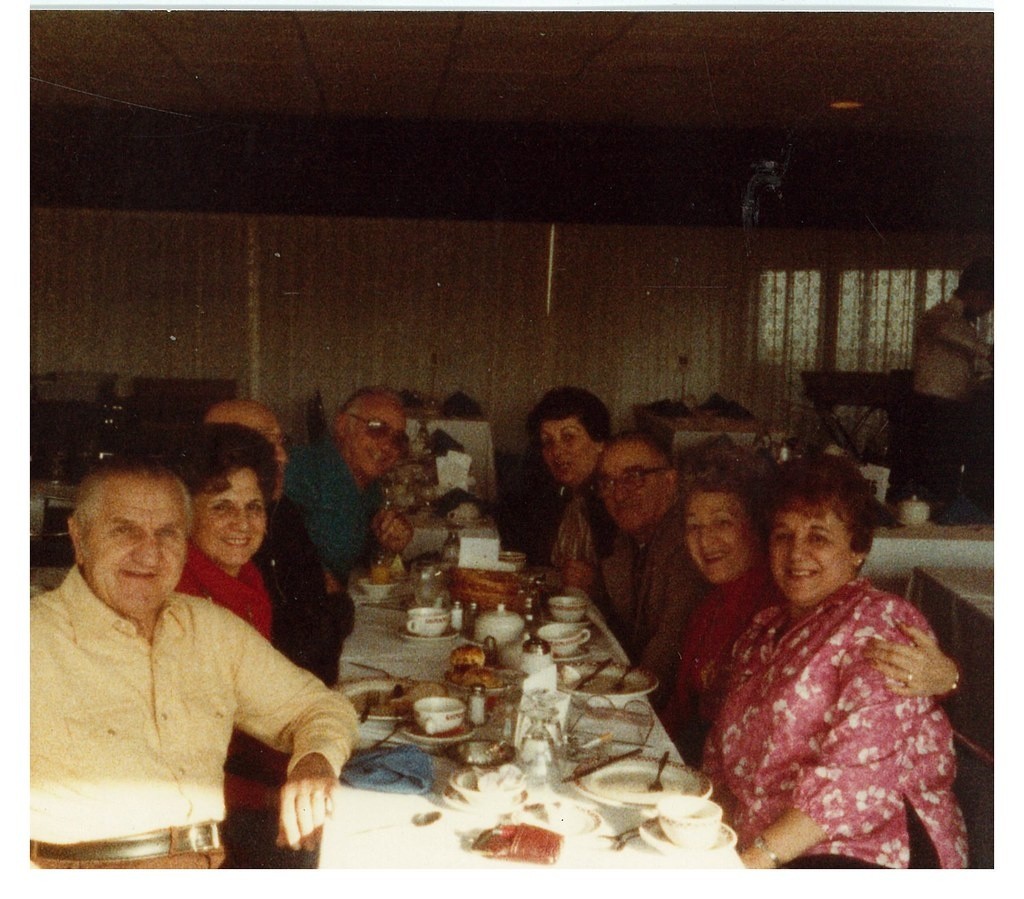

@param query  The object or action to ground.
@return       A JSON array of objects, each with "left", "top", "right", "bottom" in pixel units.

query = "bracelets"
[
  {"left": 949, "top": 671, "right": 960, "bottom": 690},
  {"left": 751, "top": 835, "right": 780, "bottom": 867},
  {"left": 373, "top": 549, "right": 393, "bottom": 567}
]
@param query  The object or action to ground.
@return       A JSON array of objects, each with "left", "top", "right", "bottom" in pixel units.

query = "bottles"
[
  {"left": 442, "top": 532, "right": 460, "bottom": 569},
  {"left": 451, "top": 574, "right": 543, "bottom": 630},
  {"left": 468, "top": 683, "right": 486, "bottom": 728}
]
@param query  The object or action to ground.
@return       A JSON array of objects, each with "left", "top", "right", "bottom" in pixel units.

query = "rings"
[
  {"left": 297, "top": 807, "right": 312, "bottom": 812},
  {"left": 906, "top": 672, "right": 913, "bottom": 683}
]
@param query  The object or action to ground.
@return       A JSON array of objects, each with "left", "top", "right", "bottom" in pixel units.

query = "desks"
[
  {"left": 858, "top": 514, "right": 994, "bottom": 594},
  {"left": 402, "top": 405, "right": 498, "bottom": 506},
  {"left": 913, "top": 565, "right": 995, "bottom": 720},
  {"left": 637, "top": 404, "right": 758, "bottom": 472}
]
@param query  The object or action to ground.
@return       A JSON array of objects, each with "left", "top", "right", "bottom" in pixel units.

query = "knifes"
[
  {"left": 573, "top": 657, "right": 614, "bottom": 689},
  {"left": 561, "top": 748, "right": 643, "bottom": 783}
]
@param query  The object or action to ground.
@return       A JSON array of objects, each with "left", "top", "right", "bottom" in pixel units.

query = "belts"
[{"left": 30, "top": 822, "right": 223, "bottom": 861}]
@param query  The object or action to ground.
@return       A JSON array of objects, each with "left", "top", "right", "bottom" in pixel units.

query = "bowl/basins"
[{"left": 446, "top": 739, "right": 526, "bottom": 806}]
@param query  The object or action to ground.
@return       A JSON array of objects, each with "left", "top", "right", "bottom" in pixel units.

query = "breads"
[{"left": 449, "top": 644, "right": 504, "bottom": 689}]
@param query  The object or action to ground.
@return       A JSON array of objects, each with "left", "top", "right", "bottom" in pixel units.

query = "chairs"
[
  {"left": 304, "top": 390, "right": 331, "bottom": 444},
  {"left": 800, "top": 371, "right": 893, "bottom": 466}
]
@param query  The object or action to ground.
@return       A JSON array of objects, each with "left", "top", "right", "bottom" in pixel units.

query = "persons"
[
  {"left": 30, "top": 386, "right": 412, "bottom": 869},
  {"left": 534, "top": 386, "right": 979, "bottom": 869}
]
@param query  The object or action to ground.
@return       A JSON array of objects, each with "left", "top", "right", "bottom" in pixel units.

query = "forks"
[
  {"left": 359, "top": 691, "right": 380, "bottom": 724},
  {"left": 650, "top": 750, "right": 670, "bottom": 793}
]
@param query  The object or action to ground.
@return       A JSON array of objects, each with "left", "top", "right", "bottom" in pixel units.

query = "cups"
[
  {"left": 413, "top": 695, "right": 465, "bottom": 735},
  {"left": 406, "top": 608, "right": 451, "bottom": 636},
  {"left": 499, "top": 551, "right": 528, "bottom": 571},
  {"left": 358, "top": 561, "right": 447, "bottom": 607},
  {"left": 536, "top": 596, "right": 593, "bottom": 656},
  {"left": 659, "top": 795, "right": 724, "bottom": 848},
  {"left": 898, "top": 493, "right": 930, "bottom": 525}
]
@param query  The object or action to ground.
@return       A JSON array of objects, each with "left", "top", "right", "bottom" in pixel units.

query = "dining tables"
[
  {"left": 318, "top": 565, "right": 746, "bottom": 869},
  {"left": 383, "top": 512, "right": 499, "bottom": 559}
]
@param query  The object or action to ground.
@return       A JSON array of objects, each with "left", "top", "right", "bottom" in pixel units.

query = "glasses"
[
  {"left": 592, "top": 466, "right": 669, "bottom": 500},
  {"left": 348, "top": 413, "right": 410, "bottom": 451}
]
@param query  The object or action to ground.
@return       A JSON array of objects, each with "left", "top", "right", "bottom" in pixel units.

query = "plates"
[
  {"left": 441, "top": 786, "right": 602, "bottom": 839},
  {"left": 572, "top": 753, "right": 714, "bottom": 811},
  {"left": 638, "top": 815, "right": 738, "bottom": 860},
  {"left": 331, "top": 666, "right": 528, "bottom": 746},
  {"left": 397, "top": 625, "right": 530, "bottom": 650},
  {"left": 539, "top": 617, "right": 659, "bottom": 698}
]
